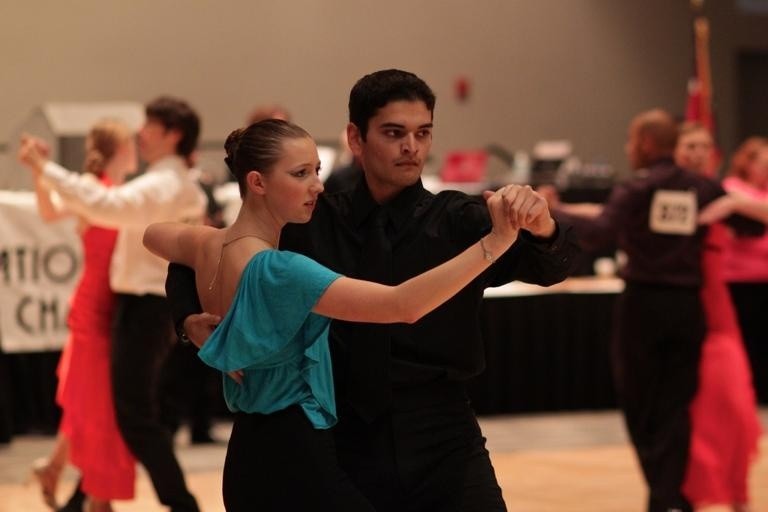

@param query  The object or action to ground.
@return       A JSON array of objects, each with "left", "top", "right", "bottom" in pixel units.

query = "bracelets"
[{"left": 479, "top": 239, "right": 496, "bottom": 265}]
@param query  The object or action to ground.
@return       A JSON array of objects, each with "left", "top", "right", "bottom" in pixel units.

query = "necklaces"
[{"left": 208, "top": 227, "right": 275, "bottom": 295}]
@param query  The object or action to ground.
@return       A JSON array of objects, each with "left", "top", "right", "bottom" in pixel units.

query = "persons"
[
  {"left": 535, "top": 109, "right": 768, "bottom": 512},
  {"left": 143, "top": 118, "right": 519, "bottom": 512},
  {"left": 17, "top": 96, "right": 209, "bottom": 512},
  {"left": 23, "top": 117, "right": 138, "bottom": 512},
  {"left": 165, "top": 69, "right": 578, "bottom": 512}
]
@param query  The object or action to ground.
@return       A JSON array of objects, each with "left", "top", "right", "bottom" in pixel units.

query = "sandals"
[{"left": 33, "top": 460, "right": 61, "bottom": 512}]
